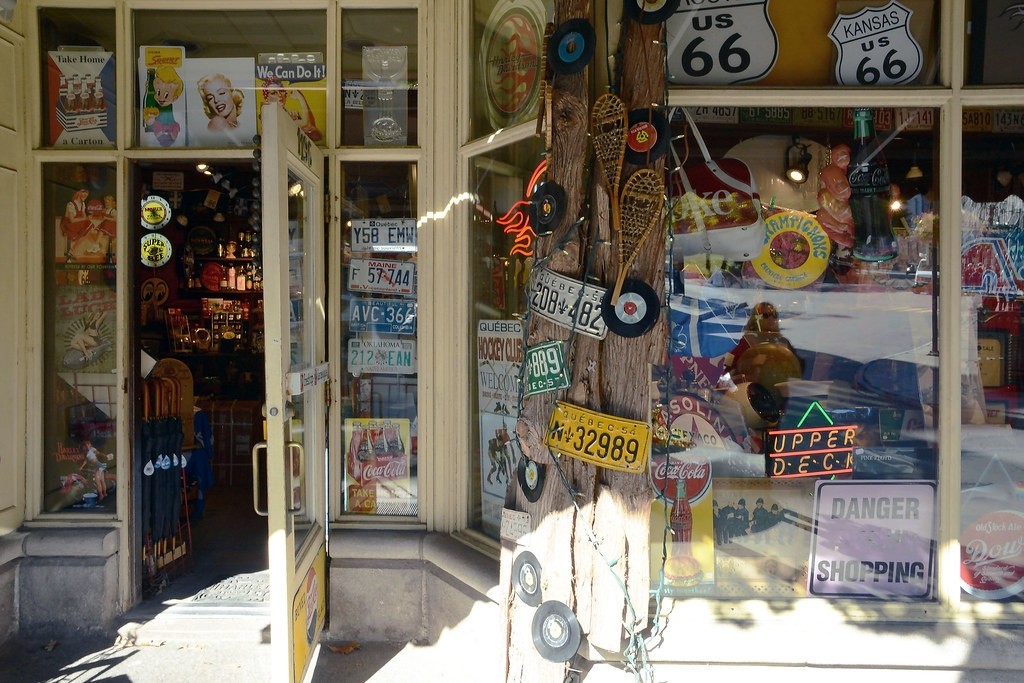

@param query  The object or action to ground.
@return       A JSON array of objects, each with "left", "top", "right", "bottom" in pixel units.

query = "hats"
[
  {"left": 737, "top": 498, "right": 746, "bottom": 505},
  {"left": 713, "top": 499, "right": 718, "bottom": 507},
  {"left": 770, "top": 504, "right": 778, "bottom": 510},
  {"left": 756, "top": 498, "right": 764, "bottom": 504}
]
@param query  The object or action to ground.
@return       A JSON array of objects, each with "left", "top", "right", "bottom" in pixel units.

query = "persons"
[
  {"left": 712, "top": 498, "right": 796, "bottom": 545},
  {"left": 196, "top": 74, "right": 244, "bottom": 132},
  {"left": 487, "top": 423, "right": 516, "bottom": 488},
  {"left": 719, "top": 301, "right": 806, "bottom": 456},
  {"left": 79, "top": 440, "right": 112, "bottom": 503},
  {"left": 70, "top": 307, "right": 110, "bottom": 365}
]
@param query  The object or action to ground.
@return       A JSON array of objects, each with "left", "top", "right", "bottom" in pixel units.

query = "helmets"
[{"left": 734, "top": 342, "right": 802, "bottom": 406}]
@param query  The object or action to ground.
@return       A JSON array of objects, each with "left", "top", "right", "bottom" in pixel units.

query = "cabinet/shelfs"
[{"left": 131, "top": 161, "right": 267, "bottom": 400}]
